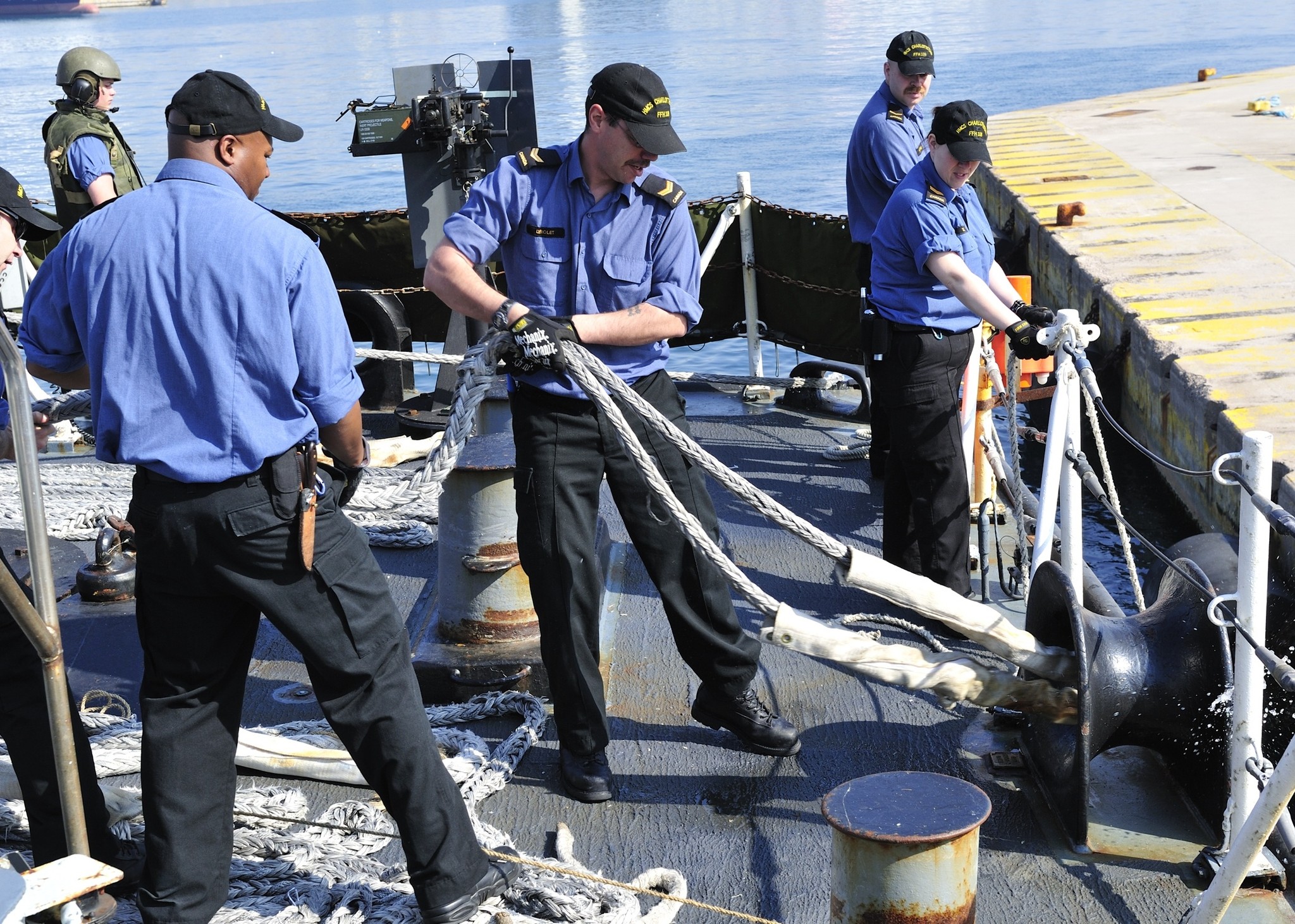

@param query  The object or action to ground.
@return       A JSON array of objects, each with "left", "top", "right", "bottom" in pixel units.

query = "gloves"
[
  {"left": 508, "top": 316, "right": 583, "bottom": 377},
  {"left": 320, "top": 434, "right": 371, "bottom": 508},
  {"left": 1005, "top": 320, "right": 1055, "bottom": 361},
  {"left": 1011, "top": 299, "right": 1055, "bottom": 327},
  {"left": 508, "top": 308, "right": 579, "bottom": 377}
]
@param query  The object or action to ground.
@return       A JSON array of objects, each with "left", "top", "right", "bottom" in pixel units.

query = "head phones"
[{"left": 67, "top": 77, "right": 99, "bottom": 106}]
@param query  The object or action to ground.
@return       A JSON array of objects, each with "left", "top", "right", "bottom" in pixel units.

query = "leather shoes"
[
  {"left": 420, "top": 845, "right": 521, "bottom": 924},
  {"left": 560, "top": 741, "right": 614, "bottom": 801},
  {"left": 103, "top": 838, "right": 147, "bottom": 896},
  {"left": 692, "top": 681, "right": 801, "bottom": 756}
]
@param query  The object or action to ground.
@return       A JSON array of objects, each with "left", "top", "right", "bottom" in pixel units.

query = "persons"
[
  {"left": 40, "top": 47, "right": 148, "bottom": 239},
  {"left": 422, "top": 62, "right": 805, "bottom": 804},
  {"left": 15, "top": 69, "right": 523, "bottom": 923},
  {"left": 870, "top": 98, "right": 1060, "bottom": 639},
  {"left": 845, "top": 31, "right": 932, "bottom": 479}
]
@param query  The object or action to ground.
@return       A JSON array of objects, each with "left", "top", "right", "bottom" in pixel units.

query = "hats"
[
  {"left": 587, "top": 62, "right": 687, "bottom": 155},
  {"left": 886, "top": 30, "right": 936, "bottom": 78},
  {"left": 931, "top": 100, "right": 992, "bottom": 167},
  {"left": 0, "top": 166, "right": 65, "bottom": 242},
  {"left": 164, "top": 69, "right": 304, "bottom": 143}
]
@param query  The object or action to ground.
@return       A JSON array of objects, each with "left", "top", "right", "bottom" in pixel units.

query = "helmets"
[{"left": 55, "top": 46, "right": 121, "bottom": 86}]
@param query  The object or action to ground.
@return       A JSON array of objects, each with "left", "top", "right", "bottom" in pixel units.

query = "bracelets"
[{"left": 1010, "top": 300, "right": 1025, "bottom": 314}]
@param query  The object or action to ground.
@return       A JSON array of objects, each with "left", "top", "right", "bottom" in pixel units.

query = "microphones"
[{"left": 91, "top": 104, "right": 120, "bottom": 113}]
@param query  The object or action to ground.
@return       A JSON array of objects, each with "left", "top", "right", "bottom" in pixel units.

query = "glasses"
[
  {"left": 602, "top": 107, "right": 642, "bottom": 148},
  {"left": 0, "top": 206, "right": 28, "bottom": 241}
]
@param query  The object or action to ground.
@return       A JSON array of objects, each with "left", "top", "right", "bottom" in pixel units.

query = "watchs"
[{"left": 491, "top": 298, "right": 516, "bottom": 331}]
